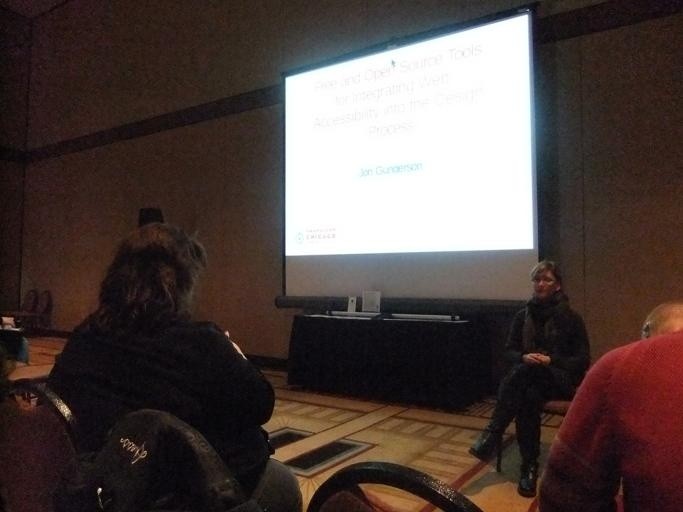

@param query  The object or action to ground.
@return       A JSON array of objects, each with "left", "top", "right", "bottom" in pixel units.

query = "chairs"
[
  {"left": 1, "top": 290, "right": 51, "bottom": 336},
  {"left": 497, "top": 398, "right": 572, "bottom": 472}
]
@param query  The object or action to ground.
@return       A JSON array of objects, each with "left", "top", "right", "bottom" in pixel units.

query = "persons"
[
  {"left": 40, "top": 222, "right": 302, "bottom": 512},
  {"left": 537, "top": 298, "right": 682, "bottom": 512},
  {"left": 467, "top": 262, "right": 589, "bottom": 497}
]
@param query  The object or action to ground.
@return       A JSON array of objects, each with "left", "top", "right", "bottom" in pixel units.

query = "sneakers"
[
  {"left": 471, "top": 429, "right": 502, "bottom": 459},
  {"left": 518, "top": 462, "right": 539, "bottom": 497}
]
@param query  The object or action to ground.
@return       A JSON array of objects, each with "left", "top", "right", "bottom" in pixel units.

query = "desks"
[{"left": 288, "top": 312, "right": 481, "bottom": 410}]
